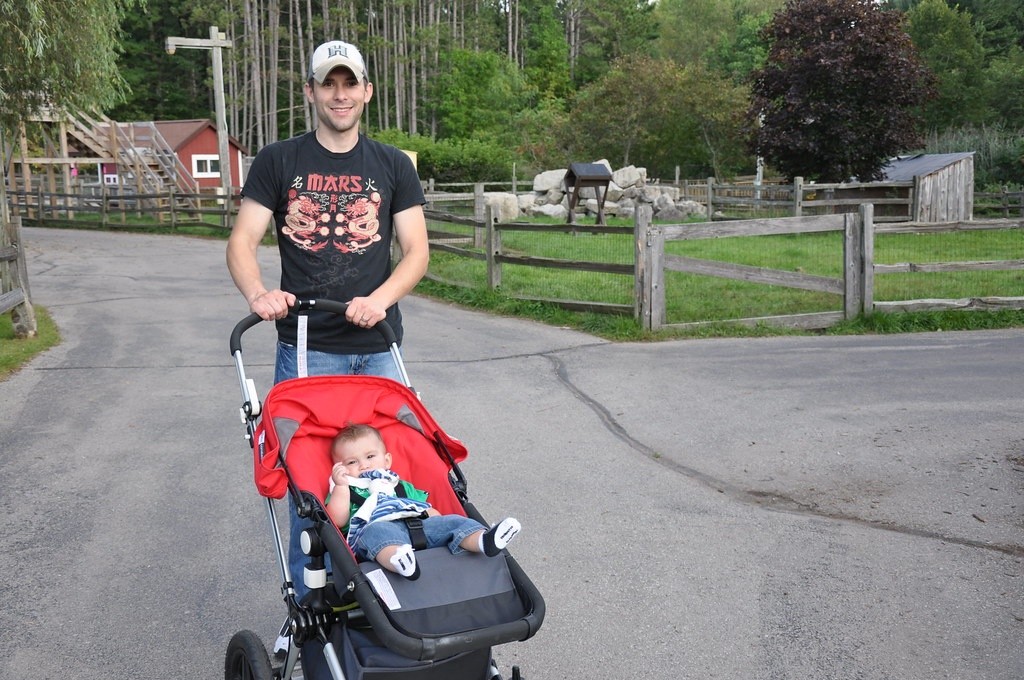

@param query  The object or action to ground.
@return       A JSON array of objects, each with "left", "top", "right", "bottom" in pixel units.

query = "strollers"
[{"left": 225, "top": 299, "right": 547, "bottom": 680}]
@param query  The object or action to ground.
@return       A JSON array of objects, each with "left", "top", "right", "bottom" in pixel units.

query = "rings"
[{"left": 360, "top": 316, "right": 370, "bottom": 323}]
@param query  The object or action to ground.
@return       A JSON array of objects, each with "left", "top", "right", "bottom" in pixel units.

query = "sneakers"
[{"left": 273, "top": 615, "right": 301, "bottom": 659}]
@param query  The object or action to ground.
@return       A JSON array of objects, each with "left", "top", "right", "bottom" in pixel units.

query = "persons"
[
  {"left": 325, "top": 421, "right": 522, "bottom": 581},
  {"left": 226, "top": 41, "right": 429, "bottom": 660}
]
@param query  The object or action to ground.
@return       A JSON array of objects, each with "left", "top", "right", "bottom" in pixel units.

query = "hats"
[{"left": 307, "top": 41, "right": 369, "bottom": 84}]
